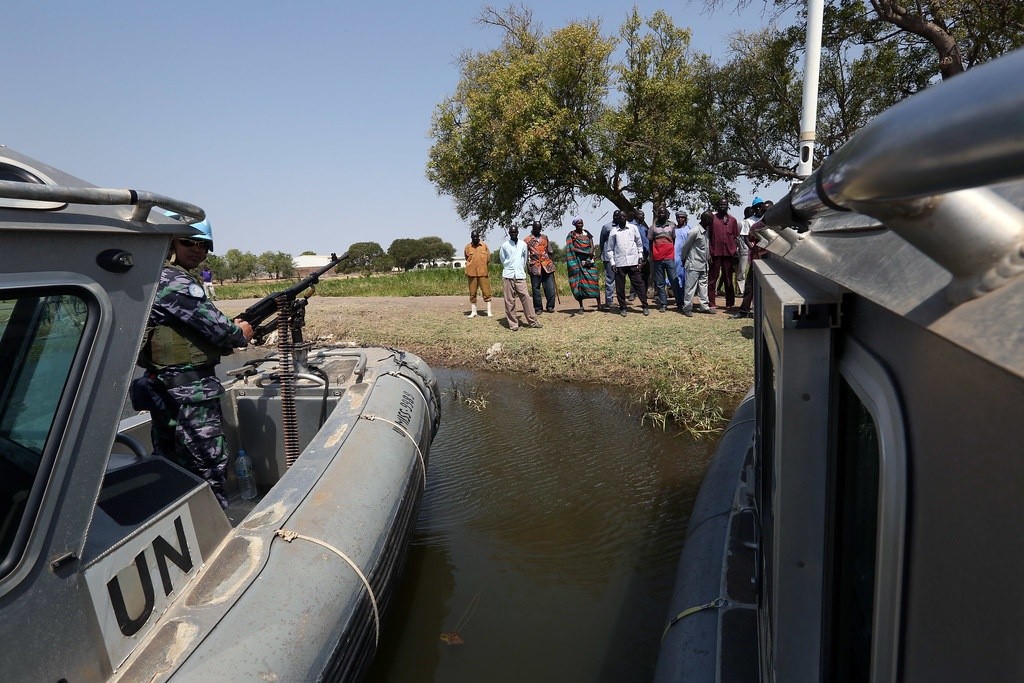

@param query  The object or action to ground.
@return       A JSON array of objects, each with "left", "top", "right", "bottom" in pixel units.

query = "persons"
[
  {"left": 199, "top": 265, "right": 219, "bottom": 301},
  {"left": 600, "top": 197, "right": 773, "bottom": 318},
  {"left": 500, "top": 225, "right": 543, "bottom": 331},
  {"left": 524, "top": 221, "right": 556, "bottom": 314},
  {"left": 566, "top": 219, "right": 604, "bottom": 313},
  {"left": 464, "top": 231, "right": 493, "bottom": 317},
  {"left": 132, "top": 211, "right": 255, "bottom": 507}
]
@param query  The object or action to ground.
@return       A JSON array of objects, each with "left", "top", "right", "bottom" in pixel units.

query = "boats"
[
  {"left": 654, "top": 46, "right": 1024, "bottom": 683},
  {"left": 0, "top": 145, "right": 441, "bottom": 683}
]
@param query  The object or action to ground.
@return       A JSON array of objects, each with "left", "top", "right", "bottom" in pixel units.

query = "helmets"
[{"left": 163, "top": 210, "right": 213, "bottom": 252}]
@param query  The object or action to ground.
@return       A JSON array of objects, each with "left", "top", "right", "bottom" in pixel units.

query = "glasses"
[{"left": 175, "top": 237, "right": 210, "bottom": 250}]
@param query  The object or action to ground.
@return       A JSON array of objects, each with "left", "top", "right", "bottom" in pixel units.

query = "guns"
[{"left": 231, "top": 250, "right": 351, "bottom": 349}]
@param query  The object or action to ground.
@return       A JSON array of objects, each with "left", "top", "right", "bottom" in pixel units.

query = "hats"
[
  {"left": 676, "top": 210, "right": 687, "bottom": 217},
  {"left": 750, "top": 196, "right": 763, "bottom": 209}
]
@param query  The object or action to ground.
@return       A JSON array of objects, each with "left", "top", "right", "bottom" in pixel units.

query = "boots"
[
  {"left": 468, "top": 303, "right": 478, "bottom": 318},
  {"left": 486, "top": 302, "right": 493, "bottom": 316}
]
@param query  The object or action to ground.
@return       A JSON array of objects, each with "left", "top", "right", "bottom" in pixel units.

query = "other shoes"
[
  {"left": 534, "top": 323, "right": 542, "bottom": 328},
  {"left": 677, "top": 305, "right": 683, "bottom": 312},
  {"left": 628, "top": 294, "right": 636, "bottom": 301},
  {"left": 547, "top": 307, "right": 555, "bottom": 312},
  {"left": 512, "top": 327, "right": 518, "bottom": 331},
  {"left": 655, "top": 298, "right": 660, "bottom": 304},
  {"left": 620, "top": 307, "right": 626, "bottom": 316},
  {"left": 685, "top": 311, "right": 693, "bottom": 317},
  {"left": 728, "top": 312, "right": 750, "bottom": 318},
  {"left": 659, "top": 304, "right": 667, "bottom": 312},
  {"left": 643, "top": 307, "right": 649, "bottom": 316},
  {"left": 724, "top": 305, "right": 733, "bottom": 310},
  {"left": 709, "top": 304, "right": 719, "bottom": 309},
  {"left": 536, "top": 310, "right": 543, "bottom": 314},
  {"left": 700, "top": 309, "right": 717, "bottom": 314},
  {"left": 607, "top": 302, "right": 614, "bottom": 308}
]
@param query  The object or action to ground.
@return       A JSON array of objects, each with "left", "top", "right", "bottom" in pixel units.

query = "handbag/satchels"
[{"left": 129, "top": 375, "right": 170, "bottom": 412}]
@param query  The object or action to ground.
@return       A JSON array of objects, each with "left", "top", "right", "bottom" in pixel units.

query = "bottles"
[{"left": 236, "top": 451, "right": 257, "bottom": 499}]
